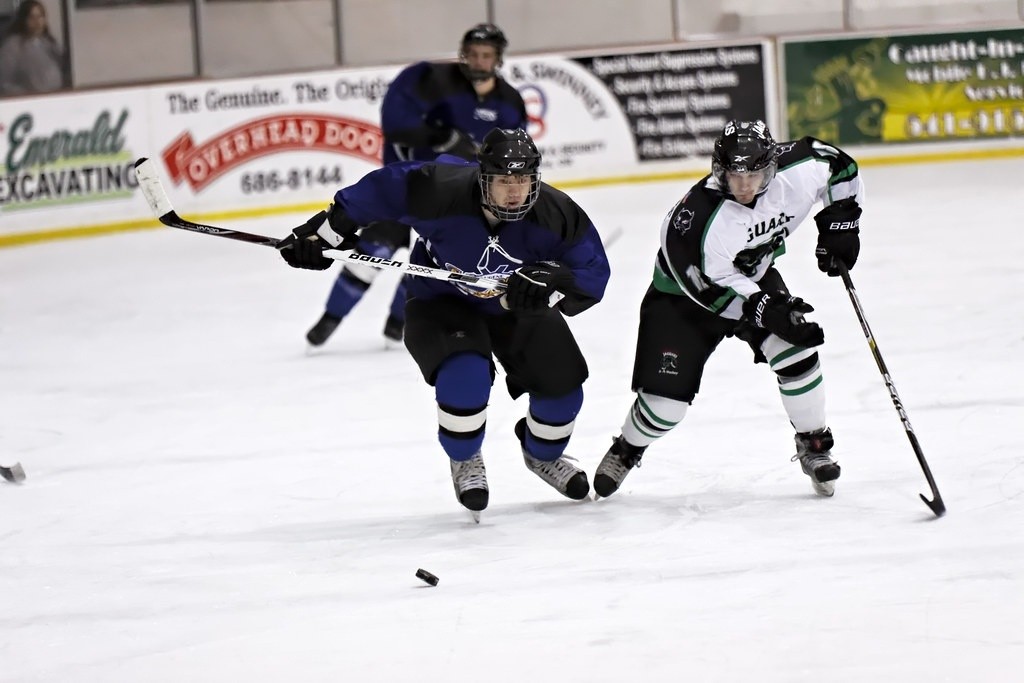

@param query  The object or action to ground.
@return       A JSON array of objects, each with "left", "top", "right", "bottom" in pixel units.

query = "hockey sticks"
[
  {"left": 832, "top": 259, "right": 946, "bottom": 518},
  {"left": 0, "top": 462, "right": 26, "bottom": 484},
  {"left": 132, "top": 157, "right": 565, "bottom": 308}
]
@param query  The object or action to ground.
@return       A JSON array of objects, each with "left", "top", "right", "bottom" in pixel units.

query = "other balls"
[{"left": 415, "top": 568, "right": 440, "bottom": 587}]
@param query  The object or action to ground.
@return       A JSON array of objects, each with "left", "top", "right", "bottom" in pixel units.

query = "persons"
[
  {"left": 594, "top": 118, "right": 861, "bottom": 503},
  {"left": 1, "top": 0, "right": 63, "bottom": 95},
  {"left": 277, "top": 126, "right": 612, "bottom": 523},
  {"left": 306, "top": 23, "right": 528, "bottom": 349}
]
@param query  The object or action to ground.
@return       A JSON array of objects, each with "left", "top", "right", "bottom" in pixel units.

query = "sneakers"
[
  {"left": 522, "top": 446, "right": 590, "bottom": 503},
  {"left": 449, "top": 450, "right": 488, "bottom": 524},
  {"left": 790, "top": 427, "right": 840, "bottom": 497},
  {"left": 593, "top": 433, "right": 647, "bottom": 500}
]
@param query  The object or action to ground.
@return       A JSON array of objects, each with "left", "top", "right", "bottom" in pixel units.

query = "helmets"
[
  {"left": 477, "top": 127, "right": 541, "bottom": 222},
  {"left": 459, "top": 23, "right": 508, "bottom": 83},
  {"left": 712, "top": 119, "right": 779, "bottom": 204}
]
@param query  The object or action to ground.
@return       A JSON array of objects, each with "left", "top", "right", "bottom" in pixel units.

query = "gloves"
[
  {"left": 275, "top": 210, "right": 349, "bottom": 271},
  {"left": 742, "top": 291, "right": 825, "bottom": 347},
  {"left": 508, "top": 257, "right": 565, "bottom": 314},
  {"left": 813, "top": 198, "right": 863, "bottom": 277}
]
